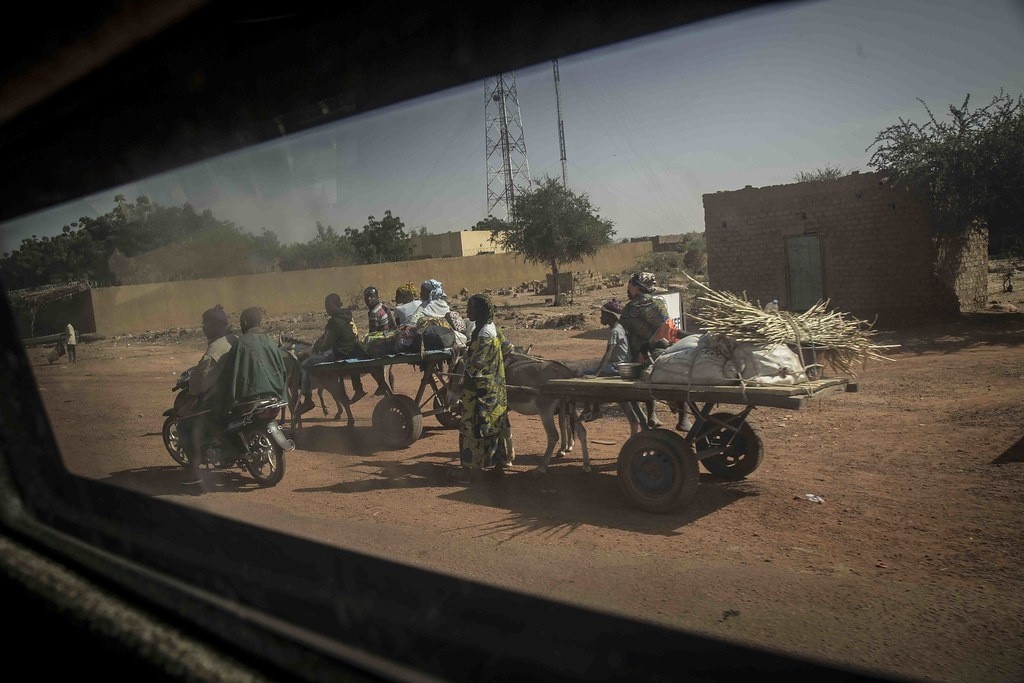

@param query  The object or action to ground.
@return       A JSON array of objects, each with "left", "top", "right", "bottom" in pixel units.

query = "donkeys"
[
  {"left": 273, "top": 330, "right": 359, "bottom": 438},
  {"left": 443, "top": 343, "right": 591, "bottom": 475}
]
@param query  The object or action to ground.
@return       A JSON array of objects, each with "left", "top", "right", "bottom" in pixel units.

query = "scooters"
[{"left": 160, "top": 362, "right": 299, "bottom": 487}]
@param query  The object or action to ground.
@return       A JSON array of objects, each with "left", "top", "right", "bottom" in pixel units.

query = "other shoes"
[
  {"left": 374, "top": 387, "right": 386, "bottom": 395},
  {"left": 482, "top": 467, "right": 504, "bottom": 480},
  {"left": 295, "top": 400, "right": 315, "bottom": 416},
  {"left": 447, "top": 468, "right": 471, "bottom": 484},
  {"left": 350, "top": 391, "right": 368, "bottom": 404},
  {"left": 180, "top": 470, "right": 202, "bottom": 485},
  {"left": 648, "top": 414, "right": 663, "bottom": 427},
  {"left": 676, "top": 417, "right": 693, "bottom": 432}
]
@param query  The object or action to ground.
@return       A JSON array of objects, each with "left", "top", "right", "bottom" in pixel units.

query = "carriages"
[
  {"left": 500, "top": 345, "right": 862, "bottom": 517},
  {"left": 267, "top": 336, "right": 468, "bottom": 449}
]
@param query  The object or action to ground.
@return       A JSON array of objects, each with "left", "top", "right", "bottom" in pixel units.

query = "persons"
[
  {"left": 618, "top": 269, "right": 699, "bottom": 432},
  {"left": 393, "top": 282, "right": 425, "bottom": 354},
  {"left": 577, "top": 301, "right": 630, "bottom": 424},
  {"left": 294, "top": 292, "right": 366, "bottom": 416},
  {"left": 202, "top": 307, "right": 288, "bottom": 465},
  {"left": 60, "top": 320, "right": 76, "bottom": 363},
  {"left": 445, "top": 295, "right": 517, "bottom": 483},
  {"left": 403, "top": 279, "right": 466, "bottom": 372},
  {"left": 360, "top": 286, "right": 397, "bottom": 396},
  {"left": 173, "top": 304, "right": 241, "bottom": 489}
]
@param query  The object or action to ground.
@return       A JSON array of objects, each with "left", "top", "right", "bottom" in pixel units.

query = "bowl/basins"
[{"left": 617, "top": 363, "right": 641, "bottom": 378}]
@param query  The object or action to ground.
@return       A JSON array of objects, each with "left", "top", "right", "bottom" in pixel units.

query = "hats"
[
  {"left": 631, "top": 270, "right": 657, "bottom": 291},
  {"left": 601, "top": 300, "right": 623, "bottom": 319},
  {"left": 240, "top": 308, "right": 261, "bottom": 328},
  {"left": 201, "top": 305, "right": 228, "bottom": 330}
]
